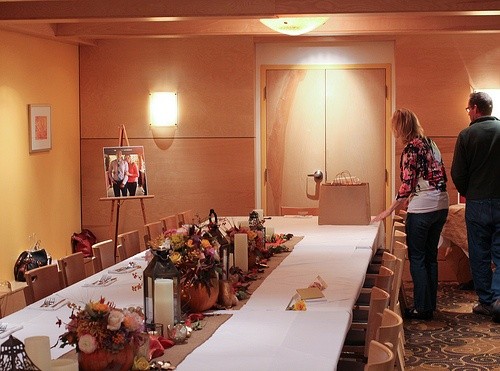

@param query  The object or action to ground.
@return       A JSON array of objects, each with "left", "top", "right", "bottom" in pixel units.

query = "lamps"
[{"left": 259, "top": 15, "right": 329, "bottom": 37}]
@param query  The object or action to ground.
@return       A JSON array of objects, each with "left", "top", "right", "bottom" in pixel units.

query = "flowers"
[
  {"left": 55, "top": 298, "right": 145, "bottom": 348},
  {"left": 144, "top": 227, "right": 229, "bottom": 284},
  {"left": 225, "top": 225, "right": 263, "bottom": 251}
]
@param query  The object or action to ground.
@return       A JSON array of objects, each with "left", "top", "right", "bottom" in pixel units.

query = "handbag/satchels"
[
  {"left": 318, "top": 170, "right": 372, "bottom": 226},
  {"left": 71, "top": 229, "right": 98, "bottom": 258},
  {"left": 14, "top": 248, "right": 53, "bottom": 281}
]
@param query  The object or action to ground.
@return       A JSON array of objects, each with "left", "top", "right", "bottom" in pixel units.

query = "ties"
[{"left": 117, "top": 159, "right": 120, "bottom": 173}]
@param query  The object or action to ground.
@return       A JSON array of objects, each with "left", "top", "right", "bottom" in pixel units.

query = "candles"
[
  {"left": 24, "top": 335, "right": 52, "bottom": 370},
  {"left": 234, "top": 232, "right": 248, "bottom": 272},
  {"left": 154, "top": 278, "right": 173, "bottom": 331},
  {"left": 264, "top": 225, "right": 274, "bottom": 236}
]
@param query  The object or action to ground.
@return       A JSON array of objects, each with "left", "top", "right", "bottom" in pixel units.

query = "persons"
[
  {"left": 451, "top": 91, "right": 500, "bottom": 322},
  {"left": 370, "top": 109, "right": 449, "bottom": 322},
  {"left": 108, "top": 149, "right": 140, "bottom": 197}
]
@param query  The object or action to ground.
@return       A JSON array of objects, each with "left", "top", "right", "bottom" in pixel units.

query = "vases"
[
  {"left": 77, "top": 335, "right": 138, "bottom": 370},
  {"left": 227, "top": 244, "right": 257, "bottom": 265},
  {"left": 181, "top": 270, "right": 221, "bottom": 311}
]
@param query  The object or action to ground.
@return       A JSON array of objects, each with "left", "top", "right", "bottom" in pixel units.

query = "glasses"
[{"left": 465, "top": 105, "right": 473, "bottom": 112}]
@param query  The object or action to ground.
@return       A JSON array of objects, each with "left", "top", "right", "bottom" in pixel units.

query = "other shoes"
[
  {"left": 406, "top": 307, "right": 434, "bottom": 320},
  {"left": 472, "top": 301, "right": 493, "bottom": 314},
  {"left": 491, "top": 300, "right": 500, "bottom": 322}
]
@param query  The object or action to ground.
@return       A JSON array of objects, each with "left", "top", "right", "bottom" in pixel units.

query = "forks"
[
  {"left": 0, "top": 322, "right": 8, "bottom": 334},
  {"left": 114, "top": 262, "right": 134, "bottom": 272},
  {"left": 40, "top": 298, "right": 55, "bottom": 307},
  {"left": 92, "top": 274, "right": 112, "bottom": 285}
]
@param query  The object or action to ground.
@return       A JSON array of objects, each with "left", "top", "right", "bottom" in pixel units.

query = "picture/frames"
[
  {"left": 28, "top": 103, "right": 51, "bottom": 153},
  {"left": 104, "top": 146, "right": 148, "bottom": 198}
]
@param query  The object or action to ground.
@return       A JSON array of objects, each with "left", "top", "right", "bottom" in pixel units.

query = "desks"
[
  {"left": 441, "top": 203, "right": 470, "bottom": 257},
  {"left": 0, "top": 214, "right": 385, "bottom": 371}
]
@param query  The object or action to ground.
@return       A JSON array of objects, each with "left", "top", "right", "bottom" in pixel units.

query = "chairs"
[
  {"left": 340, "top": 207, "right": 418, "bottom": 370},
  {"left": 0, "top": 208, "right": 194, "bottom": 318}
]
